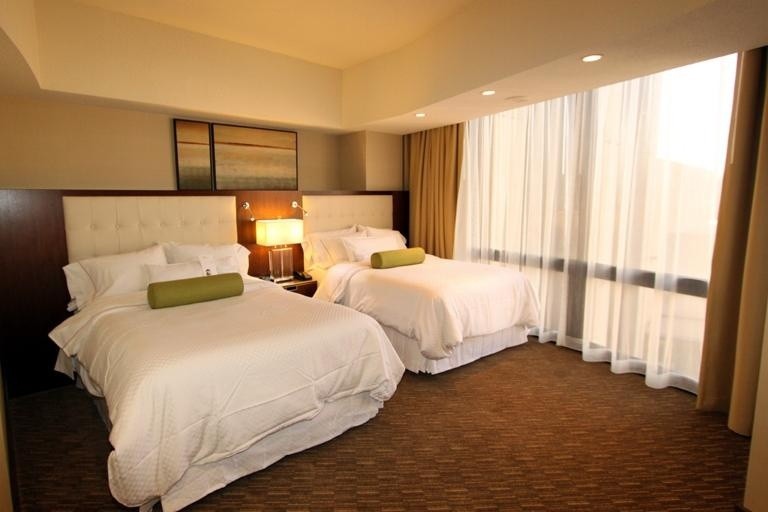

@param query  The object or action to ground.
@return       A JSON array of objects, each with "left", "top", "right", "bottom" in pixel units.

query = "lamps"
[
  {"left": 242, "top": 201, "right": 255, "bottom": 221},
  {"left": 291, "top": 201, "right": 310, "bottom": 217},
  {"left": 255, "top": 218, "right": 304, "bottom": 282}
]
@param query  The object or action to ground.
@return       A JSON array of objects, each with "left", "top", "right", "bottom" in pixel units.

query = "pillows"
[
  {"left": 62, "top": 244, "right": 167, "bottom": 309},
  {"left": 357, "top": 224, "right": 406, "bottom": 244},
  {"left": 147, "top": 272, "right": 244, "bottom": 310},
  {"left": 307, "top": 225, "right": 357, "bottom": 268},
  {"left": 144, "top": 260, "right": 207, "bottom": 286},
  {"left": 341, "top": 232, "right": 407, "bottom": 263},
  {"left": 163, "top": 242, "right": 251, "bottom": 276},
  {"left": 371, "top": 246, "right": 425, "bottom": 269}
]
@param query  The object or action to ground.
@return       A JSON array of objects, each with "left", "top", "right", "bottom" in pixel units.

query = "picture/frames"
[
  {"left": 172, "top": 119, "right": 213, "bottom": 190},
  {"left": 211, "top": 123, "right": 298, "bottom": 191}
]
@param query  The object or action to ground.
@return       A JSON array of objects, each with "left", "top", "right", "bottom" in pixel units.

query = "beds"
[
  {"left": 46, "top": 195, "right": 406, "bottom": 511},
  {"left": 302, "top": 194, "right": 541, "bottom": 376}
]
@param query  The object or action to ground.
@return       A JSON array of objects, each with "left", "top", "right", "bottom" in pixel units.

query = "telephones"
[{"left": 292, "top": 271, "right": 313, "bottom": 281}]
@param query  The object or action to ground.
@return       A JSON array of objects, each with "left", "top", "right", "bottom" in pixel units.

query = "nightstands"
[{"left": 271, "top": 277, "right": 317, "bottom": 297}]
